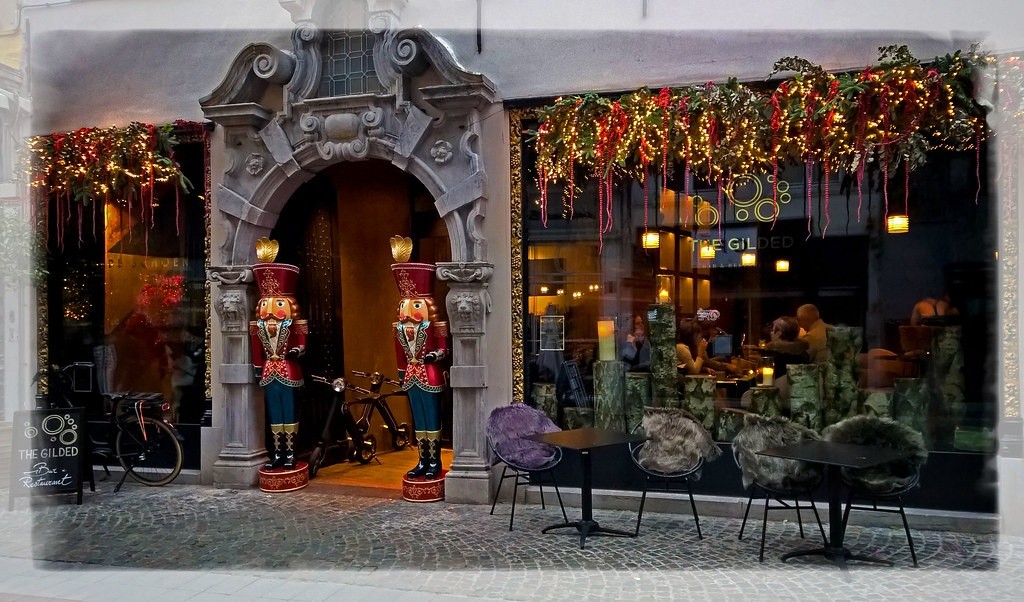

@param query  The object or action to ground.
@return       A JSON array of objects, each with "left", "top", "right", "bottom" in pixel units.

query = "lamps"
[
  {"left": 540, "top": 284, "right": 599, "bottom": 300},
  {"left": 541, "top": 216, "right": 909, "bottom": 273}
]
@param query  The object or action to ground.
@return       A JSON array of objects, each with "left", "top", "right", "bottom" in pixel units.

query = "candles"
[
  {"left": 660, "top": 290, "right": 668, "bottom": 302},
  {"left": 598, "top": 321, "right": 615, "bottom": 361},
  {"left": 763, "top": 367, "right": 773, "bottom": 385}
]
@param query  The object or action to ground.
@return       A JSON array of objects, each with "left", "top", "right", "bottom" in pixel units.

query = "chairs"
[
  {"left": 732, "top": 412, "right": 829, "bottom": 562},
  {"left": 628, "top": 407, "right": 718, "bottom": 539},
  {"left": 822, "top": 416, "right": 927, "bottom": 569},
  {"left": 486, "top": 405, "right": 570, "bottom": 532}
]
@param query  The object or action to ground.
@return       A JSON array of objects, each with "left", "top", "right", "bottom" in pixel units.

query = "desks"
[
  {"left": 755, "top": 440, "right": 913, "bottom": 584},
  {"left": 521, "top": 428, "right": 653, "bottom": 550}
]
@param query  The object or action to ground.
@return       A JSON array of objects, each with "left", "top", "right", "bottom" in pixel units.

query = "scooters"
[
  {"left": 348, "top": 370, "right": 417, "bottom": 464},
  {"left": 306, "top": 374, "right": 383, "bottom": 478}
]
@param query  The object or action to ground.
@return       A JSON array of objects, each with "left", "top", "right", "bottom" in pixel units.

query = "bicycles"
[{"left": 31, "top": 359, "right": 186, "bottom": 493}]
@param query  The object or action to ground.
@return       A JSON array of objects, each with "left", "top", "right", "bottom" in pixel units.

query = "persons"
[
  {"left": 249, "top": 236, "right": 309, "bottom": 470},
  {"left": 526, "top": 274, "right": 960, "bottom": 412},
  {"left": 388, "top": 233, "right": 451, "bottom": 479}
]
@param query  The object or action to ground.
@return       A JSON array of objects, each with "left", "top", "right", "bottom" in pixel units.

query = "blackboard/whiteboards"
[{"left": 8, "top": 406, "right": 93, "bottom": 498}]
